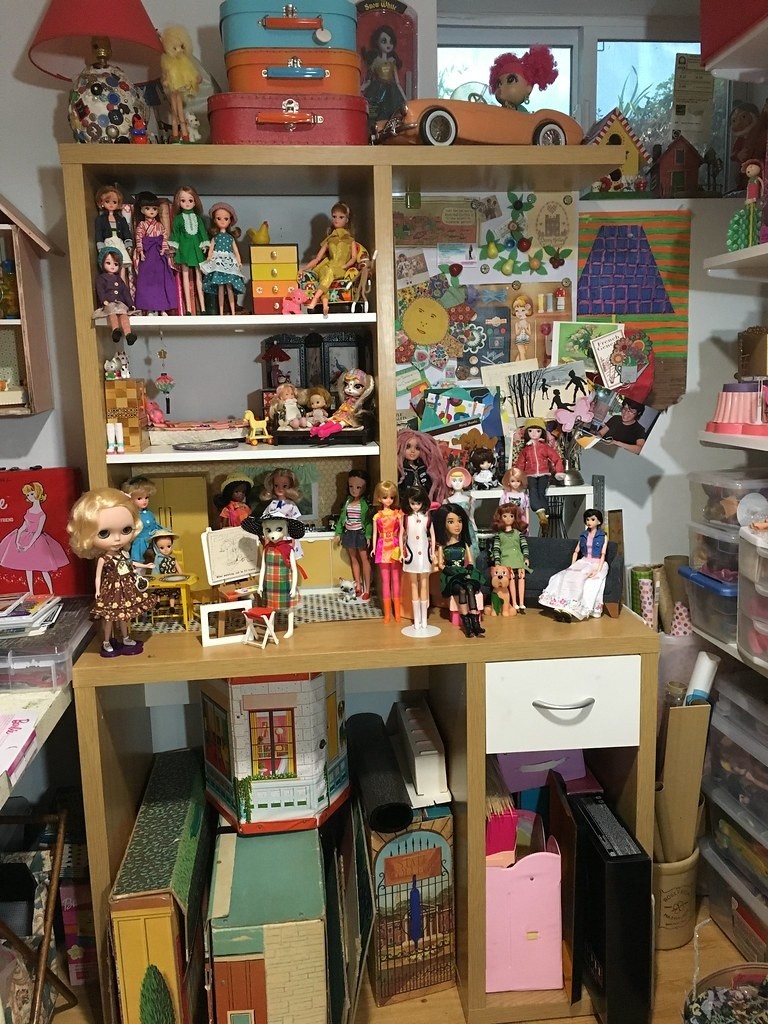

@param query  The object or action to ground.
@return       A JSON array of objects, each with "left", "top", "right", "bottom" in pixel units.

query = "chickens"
[{"left": 246, "top": 220, "right": 270, "bottom": 245}]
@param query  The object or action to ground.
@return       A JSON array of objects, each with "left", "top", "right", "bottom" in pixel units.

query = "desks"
[{"left": 149, "top": 573, "right": 197, "bottom": 630}]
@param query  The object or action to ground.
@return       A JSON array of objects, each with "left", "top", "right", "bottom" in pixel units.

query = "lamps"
[{"left": 28, "top": 0, "right": 164, "bottom": 143}]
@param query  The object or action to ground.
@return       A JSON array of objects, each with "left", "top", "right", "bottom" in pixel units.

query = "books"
[{"left": 0, "top": 593, "right": 64, "bottom": 639}]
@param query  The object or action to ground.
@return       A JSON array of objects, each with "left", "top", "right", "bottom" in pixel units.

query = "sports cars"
[{"left": 397, "top": 82, "right": 584, "bottom": 146}]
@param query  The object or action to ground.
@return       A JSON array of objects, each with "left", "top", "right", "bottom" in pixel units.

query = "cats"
[
  {"left": 115, "top": 351, "right": 131, "bottom": 379},
  {"left": 104, "top": 357, "right": 121, "bottom": 380}
]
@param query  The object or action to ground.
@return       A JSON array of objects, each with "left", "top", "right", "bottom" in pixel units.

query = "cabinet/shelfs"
[
  {"left": 688, "top": 0, "right": 768, "bottom": 678},
  {"left": 249, "top": 243, "right": 295, "bottom": 315},
  {"left": 0, "top": 195, "right": 66, "bottom": 417},
  {"left": 60, "top": 138, "right": 664, "bottom": 1024}
]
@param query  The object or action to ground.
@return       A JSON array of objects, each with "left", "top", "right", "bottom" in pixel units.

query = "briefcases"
[
  {"left": 206, "top": 91, "right": 369, "bottom": 146},
  {"left": 218, "top": 0, "right": 357, "bottom": 51},
  {"left": 223, "top": 48, "right": 362, "bottom": 97}
]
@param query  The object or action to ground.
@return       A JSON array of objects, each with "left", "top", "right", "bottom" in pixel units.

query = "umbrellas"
[{"left": 262, "top": 344, "right": 291, "bottom": 363}]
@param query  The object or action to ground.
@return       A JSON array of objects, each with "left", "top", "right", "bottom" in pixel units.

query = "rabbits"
[{"left": 146, "top": 401, "right": 166, "bottom": 428}]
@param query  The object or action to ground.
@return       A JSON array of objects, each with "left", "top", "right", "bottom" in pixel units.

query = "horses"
[{"left": 243, "top": 410, "right": 269, "bottom": 437}]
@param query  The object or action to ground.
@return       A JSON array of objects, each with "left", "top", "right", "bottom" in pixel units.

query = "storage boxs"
[
  {"left": 677, "top": 468, "right": 768, "bottom": 969},
  {"left": 208, "top": 0, "right": 371, "bottom": 145},
  {"left": 59, "top": 723, "right": 467, "bottom": 1024}
]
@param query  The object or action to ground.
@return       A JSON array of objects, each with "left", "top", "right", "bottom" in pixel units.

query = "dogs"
[
  {"left": 483, "top": 566, "right": 517, "bottom": 617},
  {"left": 185, "top": 111, "right": 203, "bottom": 143}
]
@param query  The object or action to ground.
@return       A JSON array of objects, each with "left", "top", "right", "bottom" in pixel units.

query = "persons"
[
  {"left": 729, "top": 100, "right": 768, "bottom": 190},
  {"left": 296, "top": 201, "right": 357, "bottom": 315},
  {"left": 334, "top": 417, "right": 566, "bottom": 637},
  {"left": 160, "top": 26, "right": 203, "bottom": 143},
  {"left": 597, "top": 397, "right": 645, "bottom": 454},
  {"left": 538, "top": 509, "right": 609, "bottom": 624},
  {"left": 95, "top": 184, "right": 246, "bottom": 346},
  {"left": 479, "top": 199, "right": 497, "bottom": 220},
  {"left": 66, "top": 488, "right": 157, "bottom": 658},
  {"left": 143, "top": 529, "right": 183, "bottom": 614},
  {"left": 362, "top": 25, "right": 406, "bottom": 119},
  {"left": 214, "top": 468, "right": 308, "bottom": 639},
  {"left": 741, "top": 159, "right": 764, "bottom": 200},
  {"left": 398, "top": 254, "right": 412, "bottom": 274},
  {"left": 488, "top": 44, "right": 558, "bottom": 113},
  {"left": 269, "top": 358, "right": 374, "bottom": 441},
  {"left": 122, "top": 477, "right": 163, "bottom": 569}
]
